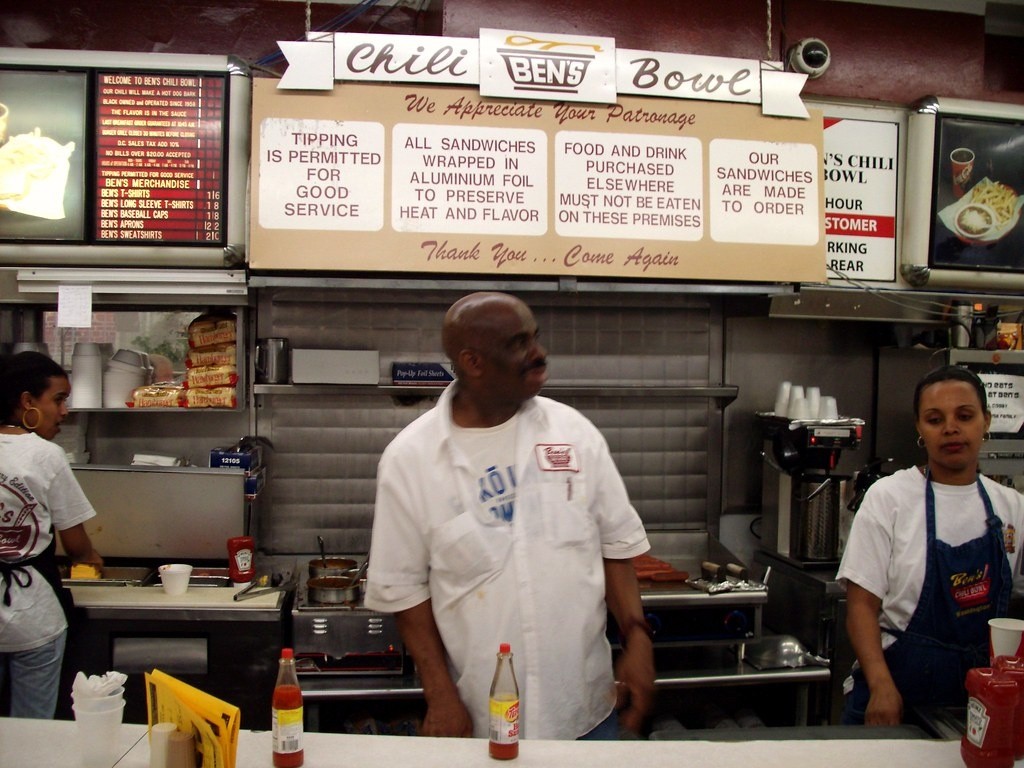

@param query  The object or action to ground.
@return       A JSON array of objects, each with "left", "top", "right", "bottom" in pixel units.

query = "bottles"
[
  {"left": 271, "top": 648, "right": 305, "bottom": 767},
  {"left": 488, "top": 643, "right": 520, "bottom": 761}
]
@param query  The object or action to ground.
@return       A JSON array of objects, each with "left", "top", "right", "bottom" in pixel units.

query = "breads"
[{"left": 129, "top": 313, "right": 238, "bottom": 409}]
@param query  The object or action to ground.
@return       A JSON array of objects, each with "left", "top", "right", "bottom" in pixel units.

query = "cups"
[
  {"left": 987, "top": 617, "right": 1024, "bottom": 667},
  {"left": 775, "top": 382, "right": 839, "bottom": 421},
  {"left": 950, "top": 147, "right": 975, "bottom": 197},
  {"left": 71, "top": 687, "right": 126, "bottom": 767},
  {"left": 255, "top": 338, "right": 288, "bottom": 384}
]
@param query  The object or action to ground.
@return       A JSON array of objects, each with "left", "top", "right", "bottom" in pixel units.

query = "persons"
[
  {"left": 834, "top": 367, "right": 1024, "bottom": 725},
  {"left": 364, "top": 291, "right": 654, "bottom": 740},
  {"left": 0, "top": 351, "right": 104, "bottom": 719}
]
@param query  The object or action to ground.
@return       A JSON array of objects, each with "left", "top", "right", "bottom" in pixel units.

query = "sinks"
[
  {"left": 58, "top": 564, "right": 157, "bottom": 588},
  {"left": 146, "top": 565, "right": 233, "bottom": 587}
]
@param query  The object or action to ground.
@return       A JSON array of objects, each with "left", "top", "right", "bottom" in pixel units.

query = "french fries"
[{"left": 970, "top": 180, "right": 1016, "bottom": 229}]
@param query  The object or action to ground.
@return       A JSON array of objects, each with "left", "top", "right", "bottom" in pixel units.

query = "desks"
[
  {"left": 71, "top": 584, "right": 286, "bottom": 625},
  {"left": 0, "top": 715, "right": 1024, "bottom": 768}
]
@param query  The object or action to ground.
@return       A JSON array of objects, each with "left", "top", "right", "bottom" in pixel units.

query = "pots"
[{"left": 306, "top": 559, "right": 369, "bottom": 603}]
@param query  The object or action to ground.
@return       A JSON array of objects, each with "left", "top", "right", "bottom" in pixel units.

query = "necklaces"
[{"left": 6, "top": 425, "right": 20, "bottom": 428}]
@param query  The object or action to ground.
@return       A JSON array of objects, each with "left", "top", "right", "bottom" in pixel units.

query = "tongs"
[{"left": 233, "top": 582, "right": 297, "bottom": 602}]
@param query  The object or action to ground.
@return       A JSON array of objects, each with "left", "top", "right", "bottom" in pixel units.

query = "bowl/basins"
[
  {"left": 954, "top": 203, "right": 995, "bottom": 238},
  {"left": 11, "top": 342, "right": 52, "bottom": 360},
  {"left": 158, "top": 564, "right": 193, "bottom": 595},
  {"left": 70, "top": 343, "right": 153, "bottom": 408}
]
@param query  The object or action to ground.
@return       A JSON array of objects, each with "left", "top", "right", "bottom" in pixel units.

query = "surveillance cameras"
[{"left": 790, "top": 37, "right": 830, "bottom": 79}]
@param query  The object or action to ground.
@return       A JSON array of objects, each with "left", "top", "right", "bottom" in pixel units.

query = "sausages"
[{"left": 632, "top": 554, "right": 689, "bottom": 581}]
